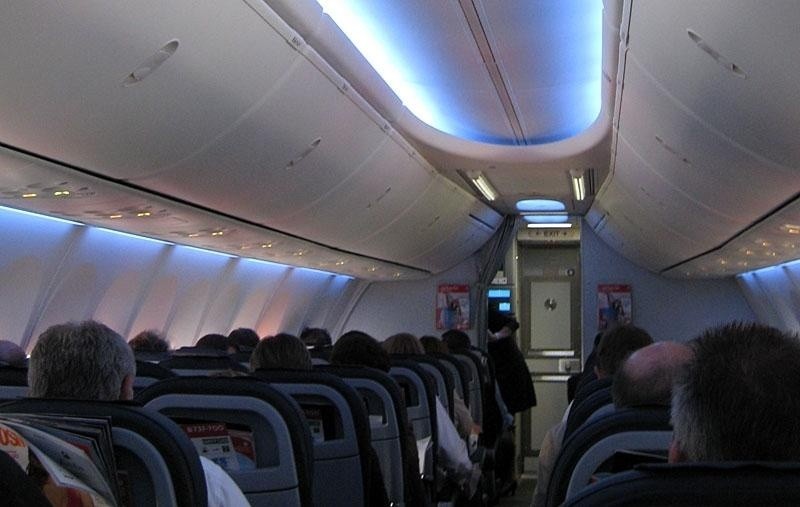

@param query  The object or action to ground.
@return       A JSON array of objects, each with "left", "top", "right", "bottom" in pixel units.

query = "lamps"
[
  {"left": 465, "top": 168, "right": 501, "bottom": 202},
  {"left": 568, "top": 165, "right": 590, "bottom": 203}
]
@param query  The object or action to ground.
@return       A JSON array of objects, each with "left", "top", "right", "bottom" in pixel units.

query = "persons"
[
  {"left": 485, "top": 302, "right": 537, "bottom": 416},
  {"left": 670, "top": 320, "right": 799, "bottom": 474},
  {"left": 530, "top": 319, "right": 708, "bottom": 504},
  {"left": 24, "top": 320, "right": 522, "bottom": 504}
]
